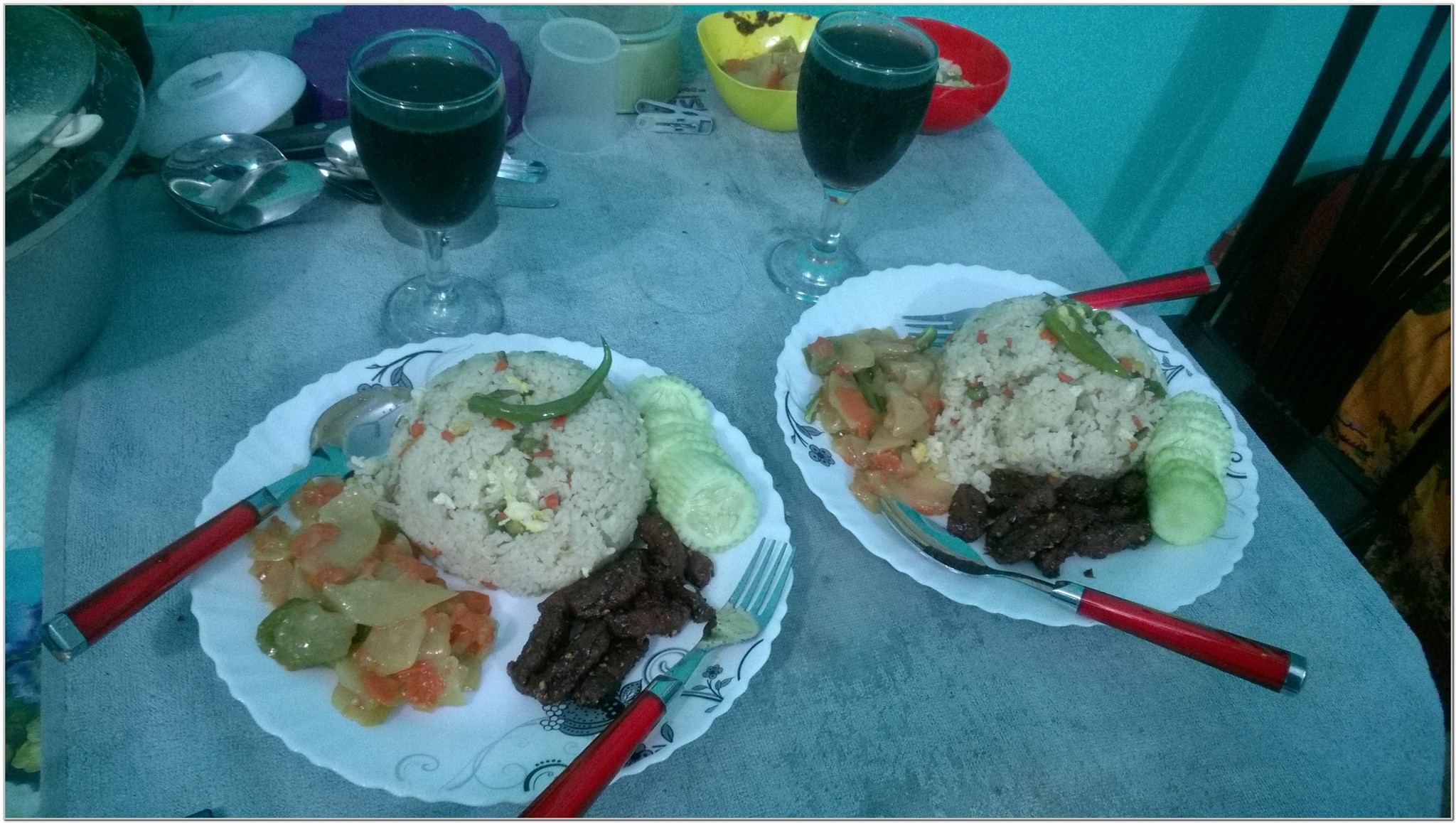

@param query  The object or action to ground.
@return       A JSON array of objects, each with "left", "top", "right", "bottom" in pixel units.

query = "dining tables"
[{"left": 6, "top": 5, "right": 1453, "bottom": 821}]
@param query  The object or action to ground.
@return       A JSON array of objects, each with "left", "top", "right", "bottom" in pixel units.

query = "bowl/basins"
[
  {"left": 897, "top": 16, "right": 1009, "bottom": 137},
  {"left": 697, "top": 11, "right": 820, "bottom": 133}
]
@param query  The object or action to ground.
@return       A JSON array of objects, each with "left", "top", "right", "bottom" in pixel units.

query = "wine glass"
[
  {"left": 345, "top": 27, "right": 510, "bottom": 347},
  {"left": 764, "top": 8, "right": 940, "bottom": 305}
]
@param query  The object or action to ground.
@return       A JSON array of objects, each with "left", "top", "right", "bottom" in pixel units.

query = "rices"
[
  {"left": 361, "top": 348, "right": 652, "bottom": 603},
  {"left": 920, "top": 289, "right": 1165, "bottom": 497}
]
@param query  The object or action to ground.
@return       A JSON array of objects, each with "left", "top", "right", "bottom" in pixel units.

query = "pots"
[{"left": 5, "top": 6, "right": 149, "bottom": 418}]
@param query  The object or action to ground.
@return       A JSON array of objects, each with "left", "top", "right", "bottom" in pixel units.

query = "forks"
[
  {"left": 514, "top": 536, "right": 797, "bottom": 818},
  {"left": 903, "top": 265, "right": 1220, "bottom": 348}
]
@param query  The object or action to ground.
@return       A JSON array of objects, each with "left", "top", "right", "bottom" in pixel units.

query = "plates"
[
  {"left": 136, "top": 50, "right": 307, "bottom": 160},
  {"left": 188, "top": 333, "right": 796, "bottom": 808},
  {"left": 774, "top": 263, "right": 1260, "bottom": 627}
]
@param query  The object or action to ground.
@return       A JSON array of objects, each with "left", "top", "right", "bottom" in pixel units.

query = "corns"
[
  {"left": 624, "top": 363, "right": 764, "bottom": 562},
  {"left": 1142, "top": 384, "right": 1235, "bottom": 549}
]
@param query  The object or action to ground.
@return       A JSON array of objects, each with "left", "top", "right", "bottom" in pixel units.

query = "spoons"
[
  {"left": 41, "top": 388, "right": 413, "bottom": 665},
  {"left": 879, "top": 497, "right": 1308, "bottom": 697},
  {"left": 158, "top": 121, "right": 562, "bottom": 237}
]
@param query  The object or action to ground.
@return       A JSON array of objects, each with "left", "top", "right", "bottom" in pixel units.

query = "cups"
[{"left": 520, "top": 16, "right": 625, "bottom": 156}]
